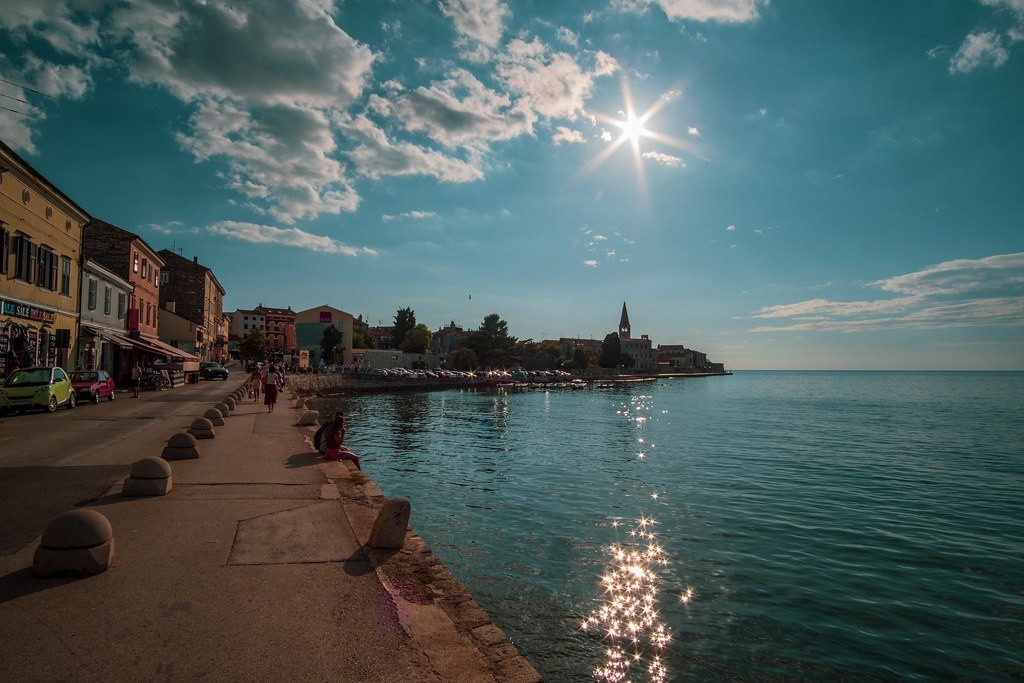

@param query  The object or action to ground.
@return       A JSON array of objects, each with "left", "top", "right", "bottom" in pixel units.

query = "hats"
[{"left": 275, "top": 366, "right": 279, "bottom": 370}]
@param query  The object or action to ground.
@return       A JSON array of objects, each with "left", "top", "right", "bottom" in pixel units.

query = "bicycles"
[{"left": 138, "top": 370, "right": 169, "bottom": 392}]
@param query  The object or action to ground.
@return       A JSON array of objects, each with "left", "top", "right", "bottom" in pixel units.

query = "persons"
[
  {"left": 318, "top": 412, "right": 346, "bottom": 454},
  {"left": 325, "top": 416, "right": 363, "bottom": 471},
  {"left": 249, "top": 358, "right": 326, "bottom": 414},
  {"left": 131, "top": 362, "right": 143, "bottom": 398}
]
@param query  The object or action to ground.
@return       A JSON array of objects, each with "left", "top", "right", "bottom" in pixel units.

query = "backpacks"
[{"left": 314, "top": 422, "right": 326, "bottom": 450}]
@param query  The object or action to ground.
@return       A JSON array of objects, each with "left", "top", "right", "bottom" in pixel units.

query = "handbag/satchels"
[{"left": 248, "top": 389, "right": 253, "bottom": 399}]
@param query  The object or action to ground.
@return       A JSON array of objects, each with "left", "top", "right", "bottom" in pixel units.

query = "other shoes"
[{"left": 131, "top": 395, "right": 139, "bottom": 398}]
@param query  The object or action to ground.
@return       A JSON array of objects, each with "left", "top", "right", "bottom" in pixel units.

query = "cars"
[
  {"left": 372, "top": 367, "right": 571, "bottom": 379},
  {"left": 64, "top": 369, "right": 117, "bottom": 404},
  {"left": 200, "top": 361, "right": 230, "bottom": 381},
  {"left": 0, "top": 366, "right": 76, "bottom": 413}
]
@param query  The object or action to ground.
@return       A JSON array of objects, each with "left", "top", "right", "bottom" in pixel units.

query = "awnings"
[{"left": 83, "top": 326, "right": 200, "bottom": 371}]
